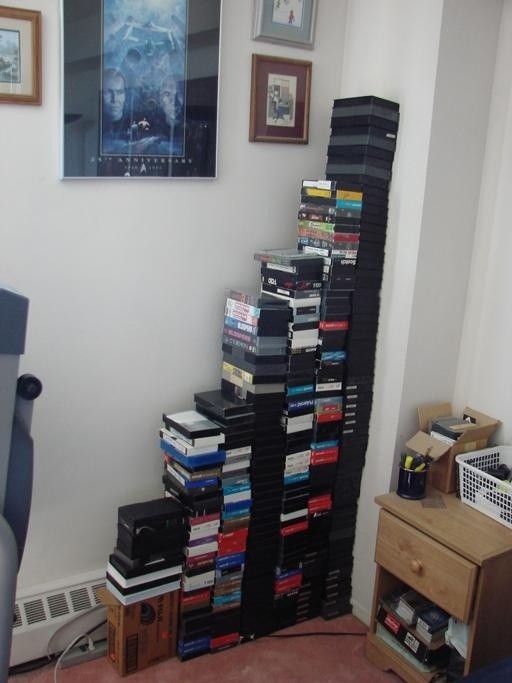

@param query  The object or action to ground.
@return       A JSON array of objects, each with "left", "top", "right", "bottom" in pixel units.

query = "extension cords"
[{"left": 60, "top": 641, "right": 107, "bottom": 668}]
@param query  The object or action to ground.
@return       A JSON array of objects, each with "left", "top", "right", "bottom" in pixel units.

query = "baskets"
[{"left": 455, "top": 445, "right": 512, "bottom": 529}]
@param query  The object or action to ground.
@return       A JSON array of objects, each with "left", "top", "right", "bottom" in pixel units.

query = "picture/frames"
[
  {"left": 0, "top": 5, "right": 42, "bottom": 106},
  {"left": 59, "top": 1, "right": 222, "bottom": 180},
  {"left": 251, "top": 0, "right": 319, "bottom": 50},
  {"left": 249, "top": 53, "right": 312, "bottom": 144}
]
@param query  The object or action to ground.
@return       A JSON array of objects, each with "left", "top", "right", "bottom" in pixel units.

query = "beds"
[{"left": 448, "top": 655, "right": 512, "bottom": 683}]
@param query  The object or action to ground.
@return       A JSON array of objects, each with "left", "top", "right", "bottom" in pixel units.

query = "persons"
[
  {"left": 152, "top": 72, "right": 186, "bottom": 156},
  {"left": 103, "top": 68, "right": 160, "bottom": 156},
  {"left": 271, "top": 89, "right": 280, "bottom": 123}
]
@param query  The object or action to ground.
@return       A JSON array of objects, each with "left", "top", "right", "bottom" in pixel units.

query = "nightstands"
[{"left": 365, "top": 482, "right": 512, "bottom": 683}]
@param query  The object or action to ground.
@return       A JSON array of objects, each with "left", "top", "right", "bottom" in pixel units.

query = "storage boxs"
[
  {"left": 406, "top": 402, "right": 499, "bottom": 494},
  {"left": 95, "top": 587, "right": 179, "bottom": 678}
]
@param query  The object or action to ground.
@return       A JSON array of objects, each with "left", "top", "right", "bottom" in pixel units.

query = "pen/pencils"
[{"left": 401, "top": 453, "right": 432, "bottom": 472}]
